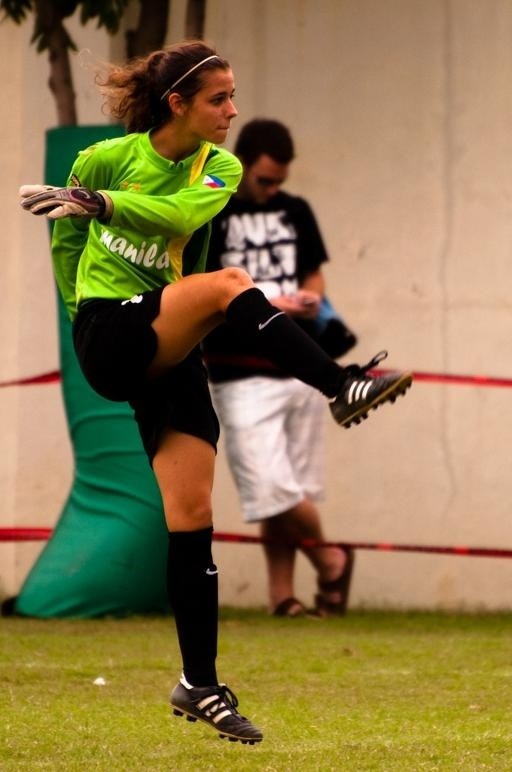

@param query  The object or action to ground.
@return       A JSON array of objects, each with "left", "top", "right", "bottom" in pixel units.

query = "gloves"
[{"left": 19, "top": 174, "right": 113, "bottom": 224}]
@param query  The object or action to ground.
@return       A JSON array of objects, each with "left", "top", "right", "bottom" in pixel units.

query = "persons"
[
  {"left": 21, "top": 42, "right": 415, "bottom": 743},
  {"left": 181, "top": 117, "right": 354, "bottom": 617}
]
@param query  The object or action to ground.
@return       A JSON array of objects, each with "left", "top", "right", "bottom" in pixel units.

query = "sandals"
[
  {"left": 273, "top": 597, "right": 314, "bottom": 617},
  {"left": 314, "top": 543, "right": 353, "bottom": 616}
]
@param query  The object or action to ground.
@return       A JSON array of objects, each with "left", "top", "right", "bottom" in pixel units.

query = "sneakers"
[
  {"left": 169, "top": 671, "right": 264, "bottom": 744},
  {"left": 329, "top": 352, "right": 411, "bottom": 428}
]
